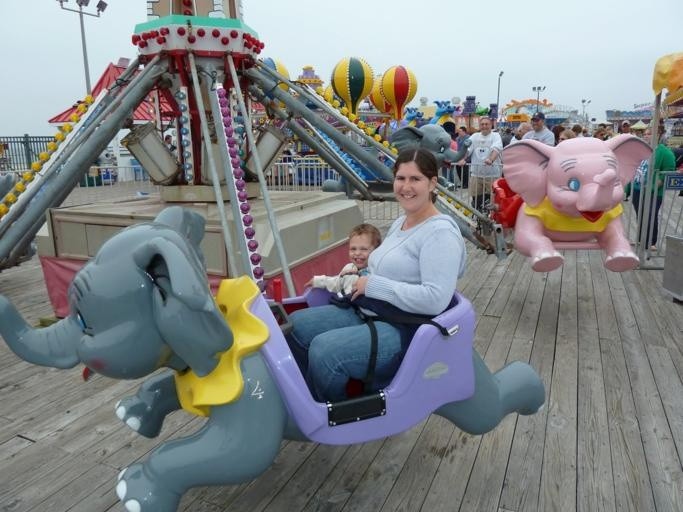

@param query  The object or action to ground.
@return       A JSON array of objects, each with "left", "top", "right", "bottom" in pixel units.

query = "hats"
[{"left": 531, "top": 112, "right": 545, "bottom": 120}]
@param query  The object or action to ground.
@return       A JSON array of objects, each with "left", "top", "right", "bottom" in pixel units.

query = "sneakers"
[
  {"left": 650, "top": 246, "right": 657, "bottom": 252},
  {"left": 629, "top": 243, "right": 640, "bottom": 247}
]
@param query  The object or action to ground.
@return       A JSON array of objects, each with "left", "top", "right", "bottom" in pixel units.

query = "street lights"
[
  {"left": 495, "top": 68, "right": 595, "bottom": 123},
  {"left": 56, "top": 0, "right": 107, "bottom": 97}
]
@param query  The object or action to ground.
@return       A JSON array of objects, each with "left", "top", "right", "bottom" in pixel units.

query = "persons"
[
  {"left": 281, "top": 148, "right": 468, "bottom": 403},
  {"left": 304, "top": 222, "right": 382, "bottom": 300},
  {"left": 438, "top": 111, "right": 676, "bottom": 252},
  {"left": 163, "top": 134, "right": 172, "bottom": 149}
]
